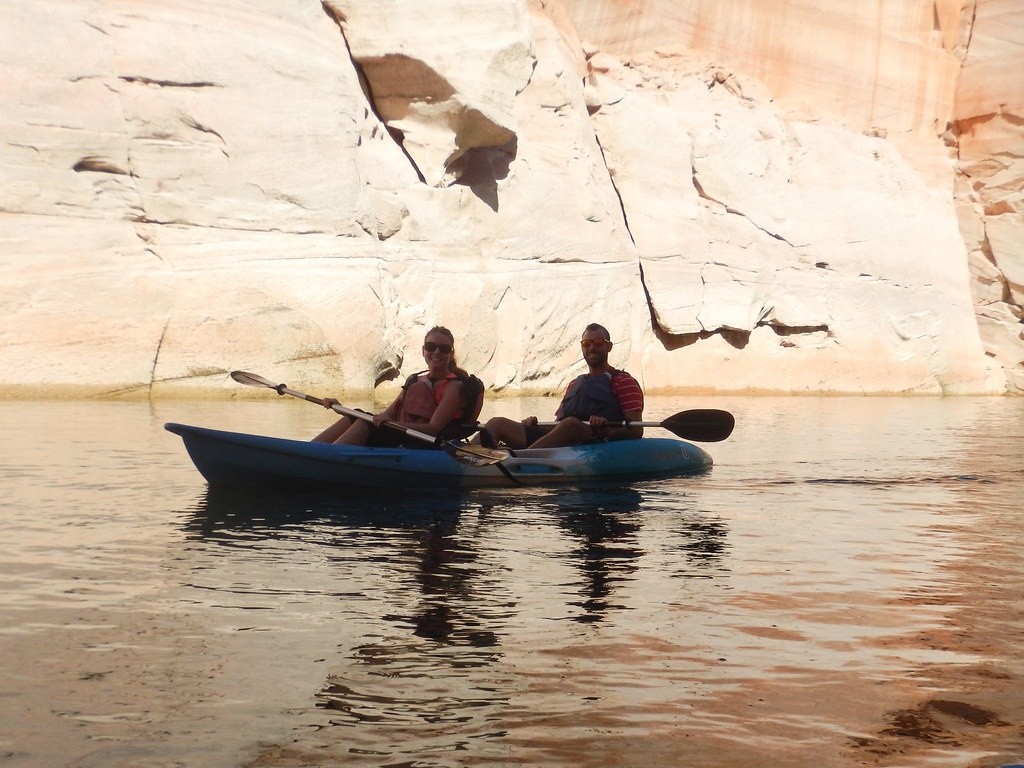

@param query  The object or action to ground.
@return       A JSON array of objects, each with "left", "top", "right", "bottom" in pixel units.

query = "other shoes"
[{"left": 480, "top": 427, "right": 497, "bottom": 450}]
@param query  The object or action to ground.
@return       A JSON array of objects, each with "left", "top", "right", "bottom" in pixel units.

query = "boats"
[{"left": 165, "top": 422, "right": 712, "bottom": 494}]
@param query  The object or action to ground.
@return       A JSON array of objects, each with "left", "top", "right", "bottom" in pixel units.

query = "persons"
[
  {"left": 469, "top": 323, "right": 643, "bottom": 448},
  {"left": 309, "top": 326, "right": 467, "bottom": 450}
]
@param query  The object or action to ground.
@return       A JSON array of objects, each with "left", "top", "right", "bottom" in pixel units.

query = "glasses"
[
  {"left": 580, "top": 338, "right": 611, "bottom": 348},
  {"left": 424, "top": 341, "right": 454, "bottom": 354}
]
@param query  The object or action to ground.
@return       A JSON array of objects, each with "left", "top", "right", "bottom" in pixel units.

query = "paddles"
[
  {"left": 228, "top": 368, "right": 512, "bottom": 469},
  {"left": 442, "top": 408, "right": 736, "bottom": 443}
]
